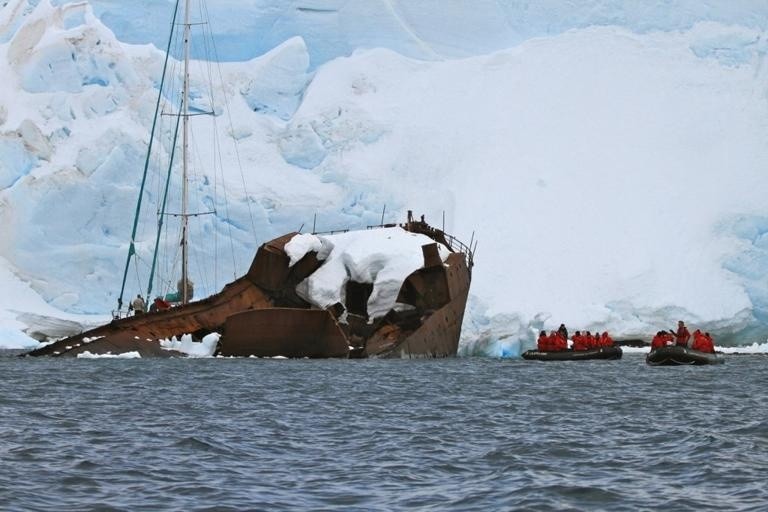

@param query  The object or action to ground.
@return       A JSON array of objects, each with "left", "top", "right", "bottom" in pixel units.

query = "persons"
[
  {"left": 538, "top": 323, "right": 614, "bottom": 352},
  {"left": 133, "top": 294, "right": 145, "bottom": 314},
  {"left": 152, "top": 296, "right": 172, "bottom": 314},
  {"left": 650, "top": 320, "right": 715, "bottom": 353},
  {"left": 149, "top": 299, "right": 158, "bottom": 313}
]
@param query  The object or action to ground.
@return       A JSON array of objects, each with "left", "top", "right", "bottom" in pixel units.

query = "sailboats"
[{"left": 18, "top": 2, "right": 474, "bottom": 359}]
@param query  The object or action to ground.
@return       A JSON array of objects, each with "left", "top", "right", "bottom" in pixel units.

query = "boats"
[
  {"left": 646, "top": 345, "right": 725, "bottom": 367},
  {"left": 519, "top": 346, "right": 623, "bottom": 362}
]
